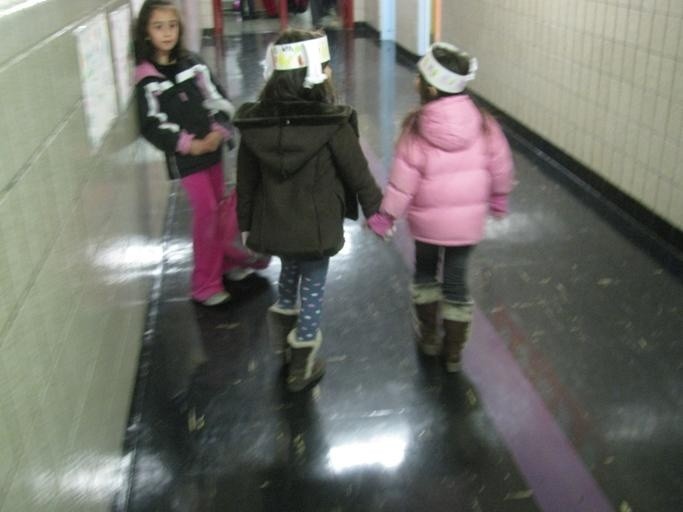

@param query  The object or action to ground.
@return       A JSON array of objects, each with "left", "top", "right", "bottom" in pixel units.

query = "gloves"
[
  {"left": 490, "top": 198, "right": 508, "bottom": 219},
  {"left": 368, "top": 213, "right": 397, "bottom": 243},
  {"left": 242, "top": 231, "right": 266, "bottom": 259}
]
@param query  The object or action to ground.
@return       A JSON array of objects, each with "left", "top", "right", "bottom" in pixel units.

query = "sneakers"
[
  {"left": 203, "top": 288, "right": 232, "bottom": 308},
  {"left": 229, "top": 267, "right": 257, "bottom": 283}
]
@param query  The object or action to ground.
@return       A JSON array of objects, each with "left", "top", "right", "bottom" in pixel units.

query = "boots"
[
  {"left": 286, "top": 325, "right": 328, "bottom": 395},
  {"left": 409, "top": 285, "right": 442, "bottom": 356},
  {"left": 266, "top": 301, "right": 301, "bottom": 377},
  {"left": 441, "top": 302, "right": 472, "bottom": 373}
]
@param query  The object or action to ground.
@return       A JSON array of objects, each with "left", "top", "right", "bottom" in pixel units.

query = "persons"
[
  {"left": 231, "top": 27, "right": 397, "bottom": 393},
  {"left": 134, "top": 0, "right": 254, "bottom": 306},
  {"left": 369, "top": 41, "right": 514, "bottom": 373}
]
